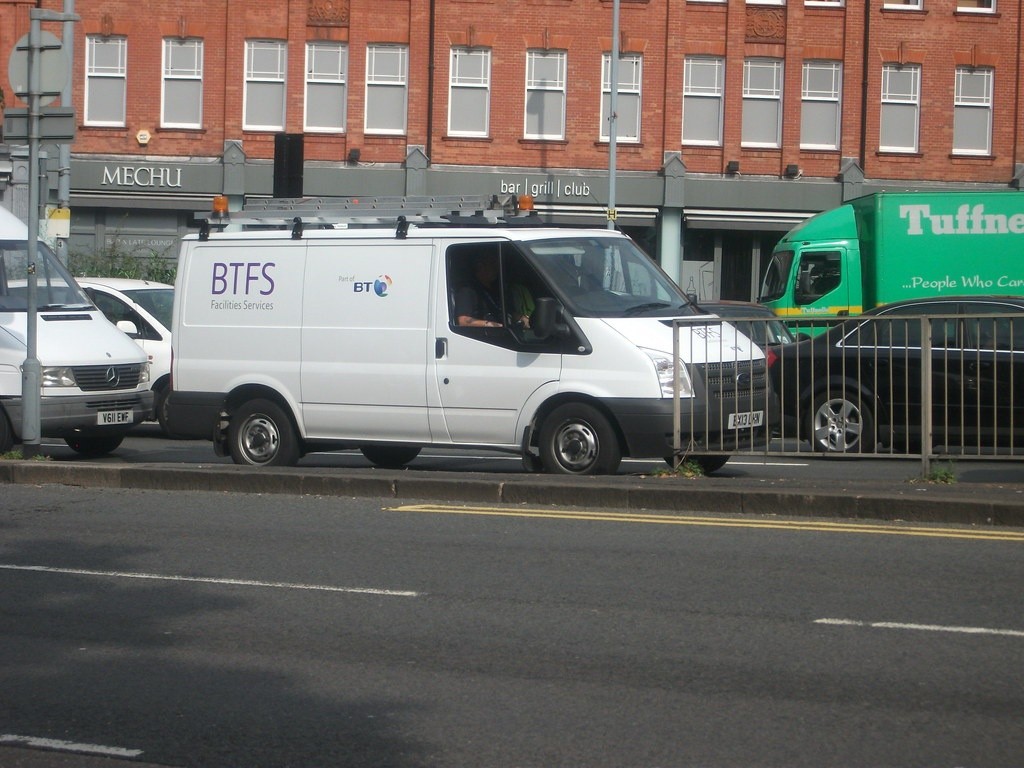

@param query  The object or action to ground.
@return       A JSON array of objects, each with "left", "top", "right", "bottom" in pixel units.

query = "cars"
[
  {"left": 694, "top": 300, "right": 812, "bottom": 352},
  {"left": 761, "top": 296, "right": 1024, "bottom": 460}
]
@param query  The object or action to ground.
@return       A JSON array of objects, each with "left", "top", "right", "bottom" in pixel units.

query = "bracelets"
[
  {"left": 484, "top": 321, "right": 488, "bottom": 326},
  {"left": 526, "top": 314, "right": 529, "bottom": 318}
]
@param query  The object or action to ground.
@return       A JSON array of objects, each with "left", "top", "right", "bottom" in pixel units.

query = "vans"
[
  {"left": 7, "top": 275, "right": 219, "bottom": 442},
  {"left": 0, "top": 204, "right": 157, "bottom": 458},
  {"left": 168, "top": 195, "right": 776, "bottom": 480}
]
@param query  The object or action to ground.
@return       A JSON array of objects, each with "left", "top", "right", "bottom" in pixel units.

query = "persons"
[{"left": 455, "top": 247, "right": 531, "bottom": 328}]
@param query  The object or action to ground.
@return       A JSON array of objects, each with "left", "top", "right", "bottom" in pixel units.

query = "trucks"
[{"left": 757, "top": 190, "right": 1024, "bottom": 341}]
